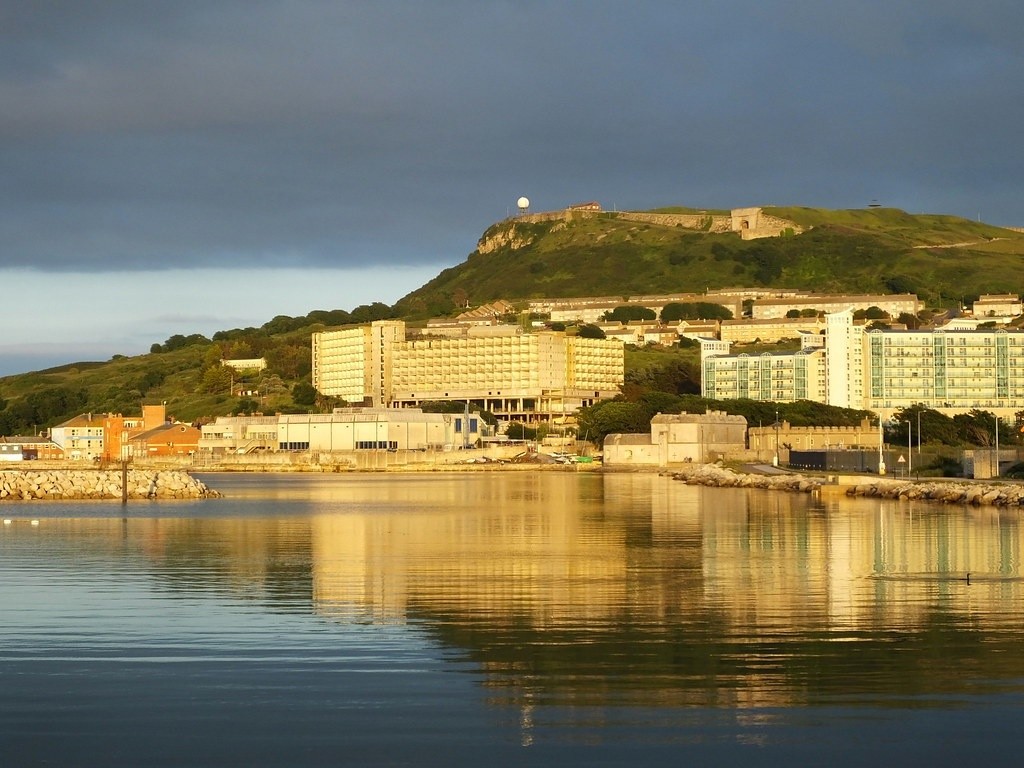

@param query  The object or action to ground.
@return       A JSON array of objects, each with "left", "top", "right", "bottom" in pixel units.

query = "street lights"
[
  {"left": 905, "top": 420, "right": 911, "bottom": 477},
  {"left": 996, "top": 416, "right": 1002, "bottom": 477},
  {"left": 918, "top": 410, "right": 927, "bottom": 454},
  {"left": 776, "top": 412, "right": 779, "bottom": 465}
]
